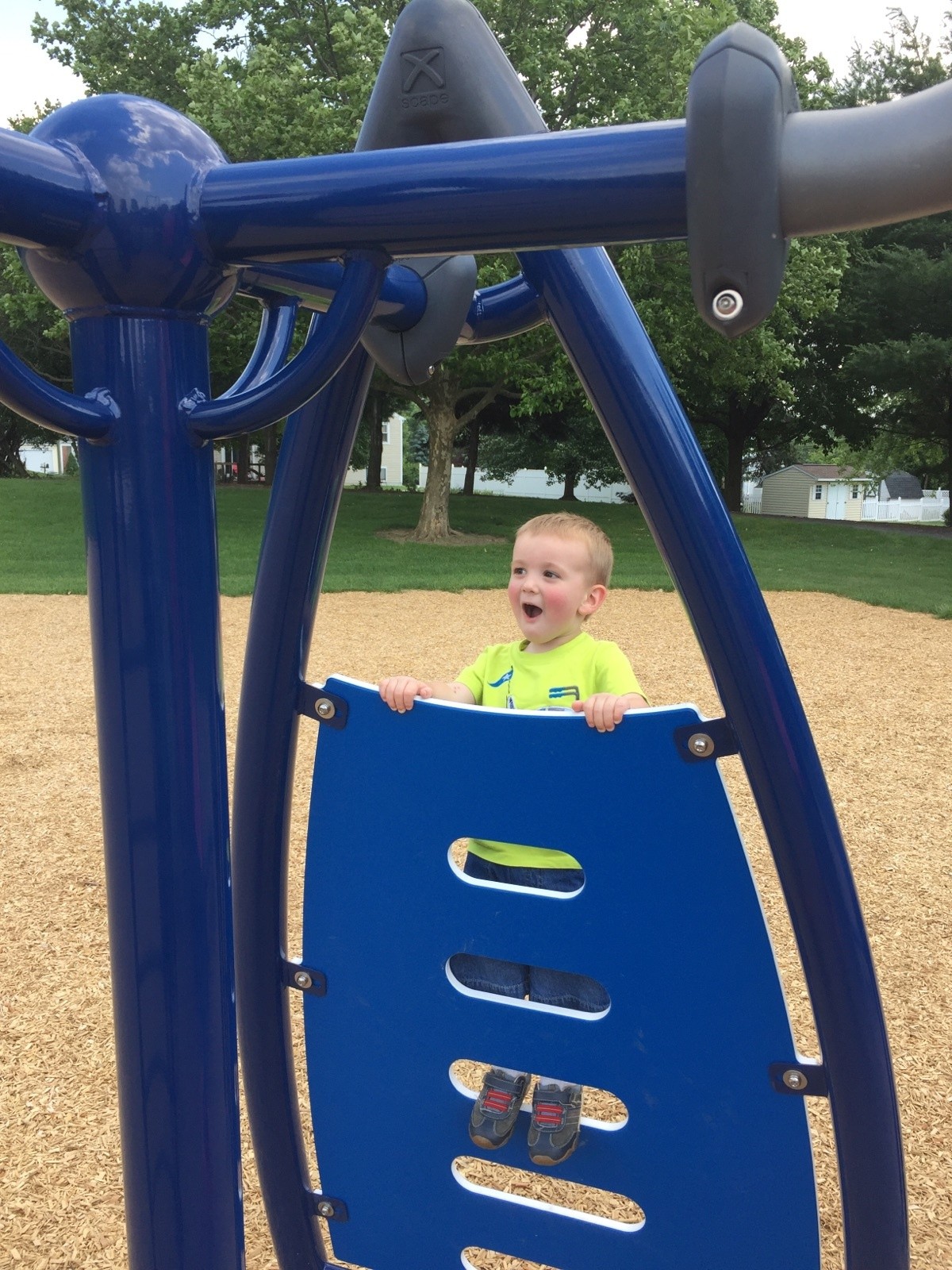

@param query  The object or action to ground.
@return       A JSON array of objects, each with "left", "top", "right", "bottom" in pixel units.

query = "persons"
[{"left": 378, "top": 511, "right": 651, "bottom": 1166}]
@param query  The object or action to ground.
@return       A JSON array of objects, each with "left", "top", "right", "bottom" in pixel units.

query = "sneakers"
[
  {"left": 527, "top": 1081, "right": 585, "bottom": 1166},
  {"left": 471, "top": 1065, "right": 532, "bottom": 1151}
]
